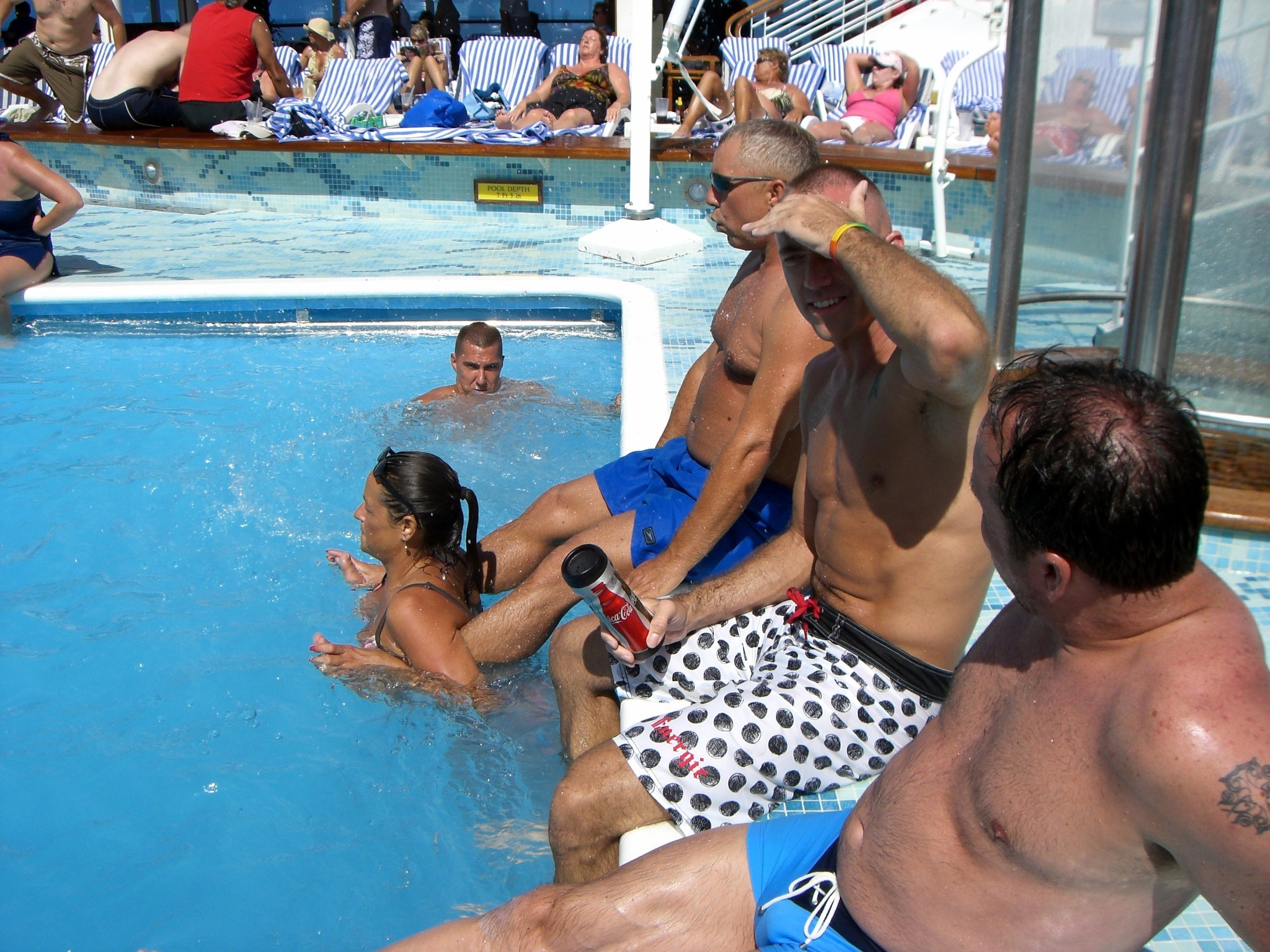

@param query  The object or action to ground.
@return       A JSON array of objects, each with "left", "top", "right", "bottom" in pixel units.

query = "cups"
[
  {"left": 245, "top": 101, "right": 262, "bottom": 124},
  {"left": 655, "top": 98, "right": 669, "bottom": 124},
  {"left": 959, "top": 112, "right": 974, "bottom": 140},
  {"left": 401, "top": 92, "right": 412, "bottom": 114}
]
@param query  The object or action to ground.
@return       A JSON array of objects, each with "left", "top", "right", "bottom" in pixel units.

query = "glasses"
[
  {"left": 410, "top": 37, "right": 426, "bottom": 44},
  {"left": 710, "top": 170, "right": 786, "bottom": 195},
  {"left": 756, "top": 57, "right": 772, "bottom": 64},
  {"left": 372, "top": 446, "right": 421, "bottom": 531},
  {"left": 1075, "top": 77, "right": 1097, "bottom": 91}
]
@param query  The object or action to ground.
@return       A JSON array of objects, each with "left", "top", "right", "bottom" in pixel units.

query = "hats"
[
  {"left": 303, "top": 18, "right": 335, "bottom": 41},
  {"left": 867, "top": 50, "right": 903, "bottom": 74}
]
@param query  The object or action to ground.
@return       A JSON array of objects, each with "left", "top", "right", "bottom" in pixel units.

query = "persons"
[
  {"left": 0, "top": 132, "right": 84, "bottom": 334},
  {"left": 411, "top": 322, "right": 505, "bottom": 403},
  {"left": 309, "top": 445, "right": 481, "bottom": 686},
  {"left": 314, "top": 118, "right": 818, "bottom": 676},
  {"left": 383, "top": 345, "right": 1270, "bottom": 952},
  {"left": 549, "top": 162, "right": 1000, "bottom": 889},
  {"left": 0, "top": 0, "right": 1238, "bottom": 178}
]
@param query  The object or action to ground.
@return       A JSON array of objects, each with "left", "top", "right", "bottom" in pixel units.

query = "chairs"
[
  {"left": 546, "top": 34, "right": 630, "bottom": 137},
  {"left": 44, "top": 43, "right": 117, "bottom": 121},
  {"left": 944, "top": 44, "right": 1007, "bottom": 130},
  {"left": 389, "top": 39, "right": 453, "bottom": 85},
  {"left": 1039, "top": 46, "right": 1139, "bottom": 172},
  {"left": 455, "top": 38, "right": 547, "bottom": 121},
  {"left": 721, "top": 36, "right": 792, "bottom": 96},
  {"left": 0, "top": 46, "right": 44, "bottom": 110},
  {"left": 813, "top": 42, "right": 882, "bottom": 115},
  {"left": 821, "top": 69, "right": 934, "bottom": 149},
  {"left": 312, "top": 57, "right": 406, "bottom": 123},
  {"left": 274, "top": 43, "right": 304, "bottom": 90},
  {"left": 737, "top": 57, "right": 826, "bottom": 128}
]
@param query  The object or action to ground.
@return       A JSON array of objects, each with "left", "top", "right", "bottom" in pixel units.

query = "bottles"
[
  {"left": 561, "top": 544, "right": 665, "bottom": 661},
  {"left": 676, "top": 97, "right": 684, "bottom": 124}
]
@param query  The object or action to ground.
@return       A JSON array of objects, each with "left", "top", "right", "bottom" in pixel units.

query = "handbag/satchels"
[
  {"left": 462, "top": 82, "right": 511, "bottom": 121},
  {"left": 399, "top": 88, "right": 471, "bottom": 132}
]
